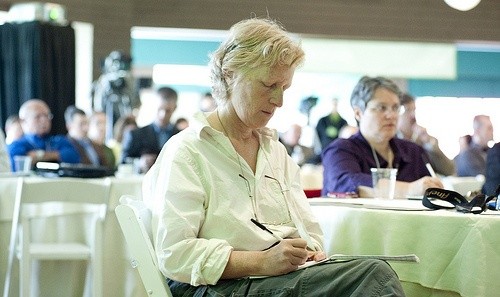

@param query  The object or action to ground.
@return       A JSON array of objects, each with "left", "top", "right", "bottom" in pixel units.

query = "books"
[{"left": 249, "top": 254, "right": 419, "bottom": 279}]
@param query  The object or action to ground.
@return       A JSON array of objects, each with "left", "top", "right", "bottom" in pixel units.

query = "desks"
[{"left": 308, "top": 198, "right": 500, "bottom": 297}]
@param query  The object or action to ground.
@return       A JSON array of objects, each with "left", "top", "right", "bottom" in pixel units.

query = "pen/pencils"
[{"left": 250, "top": 217, "right": 285, "bottom": 242}]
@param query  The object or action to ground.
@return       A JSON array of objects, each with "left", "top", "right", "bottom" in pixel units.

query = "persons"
[
  {"left": 0, "top": 76, "right": 500, "bottom": 199},
  {"left": 141, "top": 17, "right": 405, "bottom": 297}
]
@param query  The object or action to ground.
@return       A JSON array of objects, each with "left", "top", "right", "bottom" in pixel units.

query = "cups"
[
  {"left": 14, "top": 155, "right": 32, "bottom": 176},
  {"left": 371, "top": 168, "right": 397, "bottom": 199}
]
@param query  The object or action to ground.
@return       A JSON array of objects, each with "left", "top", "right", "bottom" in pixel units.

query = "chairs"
[
  {"left": 115, "top": 204, "right": 173, "bottom": 297},
  {"left": 3, "top": 177, "right": 114, "bottom": 297}
]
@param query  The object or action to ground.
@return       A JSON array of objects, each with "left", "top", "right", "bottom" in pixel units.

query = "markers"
[{"left": 421, "top": 152, "right": 438, "bottom": 178}]
